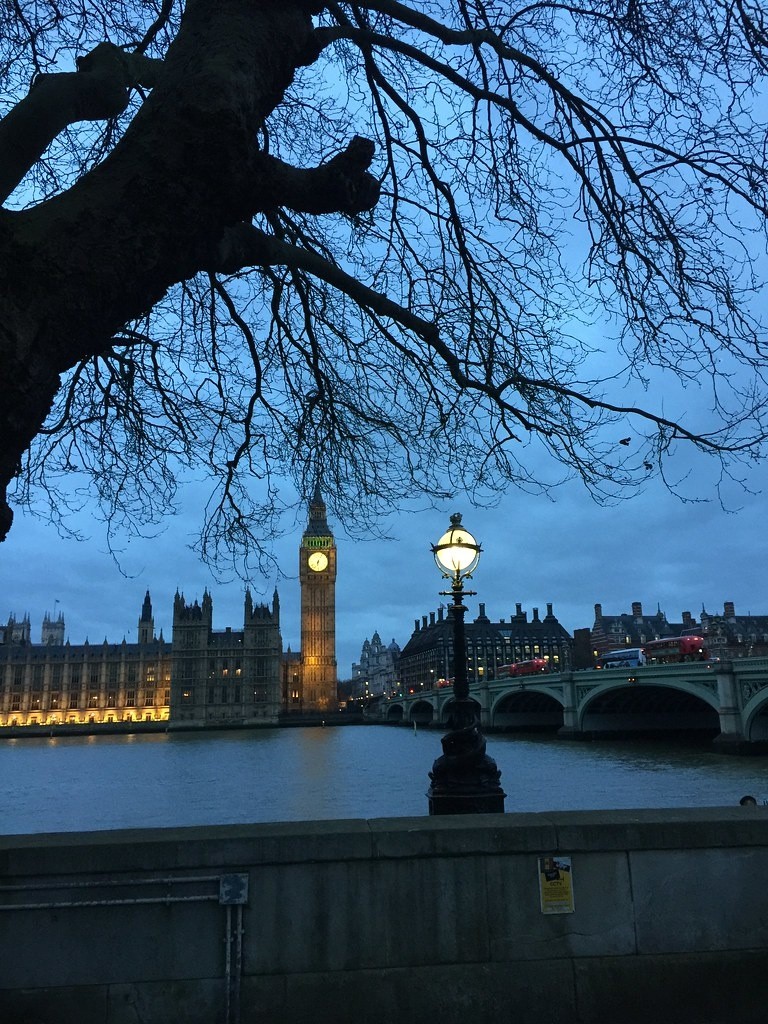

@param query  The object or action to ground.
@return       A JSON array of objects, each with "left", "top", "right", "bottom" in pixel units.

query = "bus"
[
  {"left": 644, "top": 634, "right": 709, "bottom": 665},
  {"left": 511, "top": 657, "right": 547, "bottom": 678},
  {"left": 602, "top": 647, "right": 646, "bottom": 671}
]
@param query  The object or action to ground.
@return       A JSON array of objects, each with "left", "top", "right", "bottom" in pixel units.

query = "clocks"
[{"left": 308, "top": 552, "right": 328, "bottom": 572}]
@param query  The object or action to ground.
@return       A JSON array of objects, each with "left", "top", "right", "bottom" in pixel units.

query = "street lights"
[{"left": 424, "top": 511, "right": 509, "bottom": 818}]
